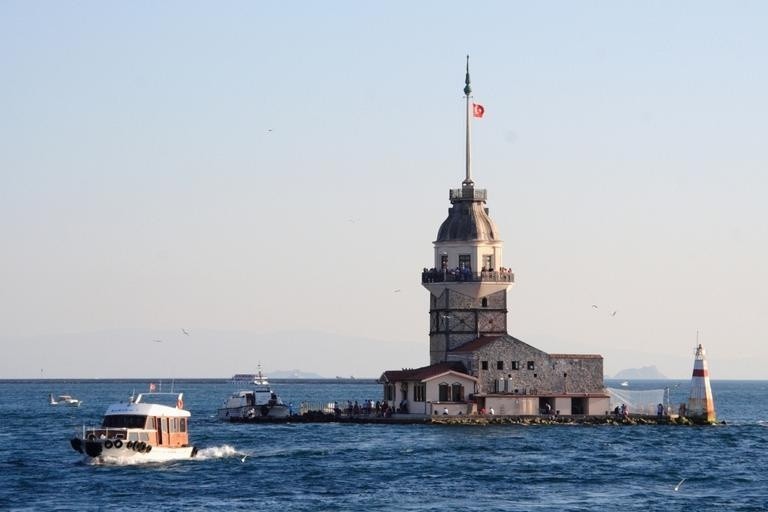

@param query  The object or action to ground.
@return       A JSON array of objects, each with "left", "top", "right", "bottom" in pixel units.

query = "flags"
[
  {"left": 150, "top": 383, "right": 155, "bottom": 391},
  {"left": 472, "top": 102, "right": 485, "bottom": 118}
]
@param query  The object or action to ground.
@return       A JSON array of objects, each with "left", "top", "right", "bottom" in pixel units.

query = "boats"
[
  {"left": 49, "top": 393, "right": 82, "bottom": 406},
  {"left": 70, "top": 391, "right": 199, "bottom": 462},
  {"left": 226, "top": 364, "right": 269, "bottom": 385},
  {"left": 217, "top": 387, "right": 289, "bottom": 422}
]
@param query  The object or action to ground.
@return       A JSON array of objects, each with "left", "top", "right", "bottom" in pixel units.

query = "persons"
[
  {"left": 615, "top": 406, "right": 620, "bottom": 414},
  {"left": 545, "top": 403, "right": 551, "bottom": 414},
  {"left": 488, "top": 408, "right": 495, "bottom": 416},
  {"left": 442, "top": 407, "right": 448, "bottom": 416},
  {"left": 621, "top": 404, "right": 627, "bottom": 413},
  {"left": 423, "top": 265, "right": 512, "bottom": 283},
  {"left": 480, "top": 407, "right": 485, "bottom": 414},
  {"left": 657, "top": 403, "right": 663, "bottom": 415},
  {"left": 333, "top": 399, "right": 408, "bottom": 417}
]
